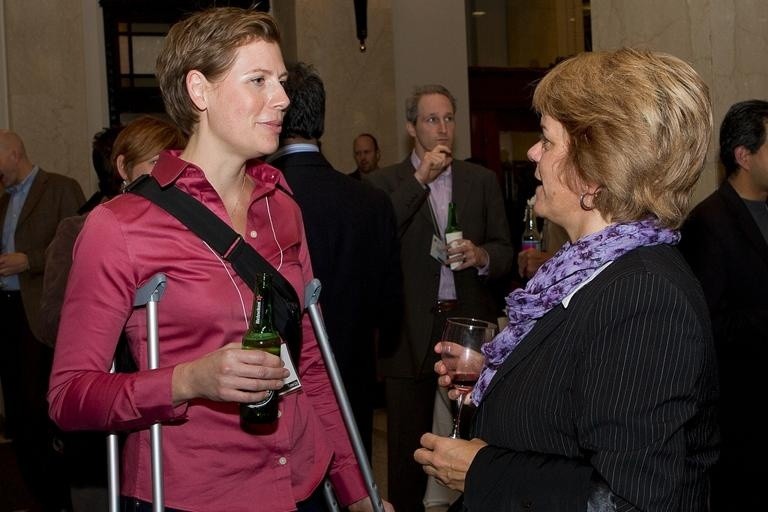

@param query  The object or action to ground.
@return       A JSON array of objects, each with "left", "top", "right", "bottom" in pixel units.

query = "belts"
[{"left": 436, "top": 299, "right": 460, "bottom": 313}]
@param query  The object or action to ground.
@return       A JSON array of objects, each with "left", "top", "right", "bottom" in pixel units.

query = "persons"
[
  {"left": 672, "top": 99, "right": 767, "bottom": 510},
  {"left": 369, "top": 84, "right": 513, "bottom": 511},
  {"left": 517, "top": 218, "right": 568, "bottom": 279},
  {"left": 38, "top": 117, "right": 186, "bottom": 510},
  {"left": 411, "top": 43, "right": 722, "bottom": 510},
  {"left": 2, "top": 130, "right": 86, "bottom": 511},
  {"left": 259, "top": 61, "right": 401, "bottom": 510},
  {"left": 45, "top": 8, "right": 397, "bottom": 511}
]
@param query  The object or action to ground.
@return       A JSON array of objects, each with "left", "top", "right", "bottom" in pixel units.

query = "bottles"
[
  {"left": 522, "top": 205, "right": 542, "bottom": 255},
  {"left": 445, "top": 201, "right": 466, "bottom": 273},
  {"left": 240, "top": 273, "right": 282, "bottom": 436}
]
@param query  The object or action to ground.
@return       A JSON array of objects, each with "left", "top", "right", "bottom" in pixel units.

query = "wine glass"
[{"left": 441, "top": 318, "right": 498, "bottom": 441}]
